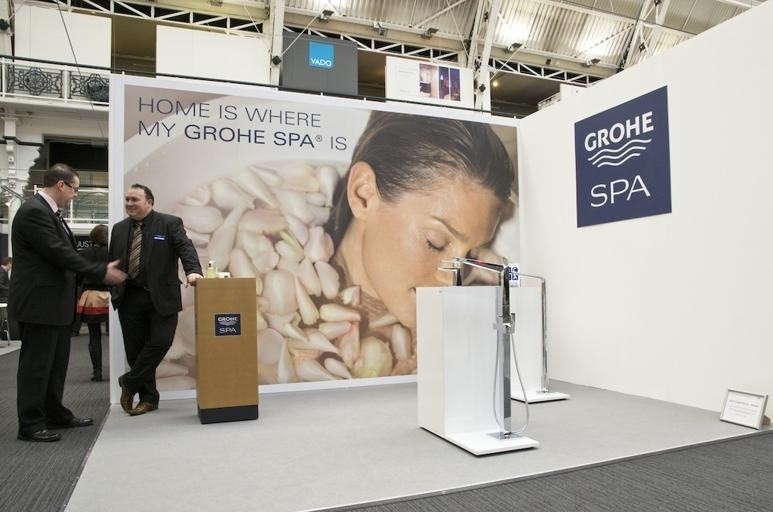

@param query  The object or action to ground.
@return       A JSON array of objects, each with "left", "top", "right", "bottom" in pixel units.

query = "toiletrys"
[{"left": 206, "top": 261, "right": 216, "bottom": 279}]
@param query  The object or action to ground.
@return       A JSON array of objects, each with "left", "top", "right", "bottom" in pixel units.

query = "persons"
[
  {"left": 105, "top": 184, "right": 203, "bottom": 416},
  {"left": 76, "top": 224, "right": 109, "bottom": 381},
  {"left": 330, "top": 110, "right": 516, "bottom": 337},
  {"left": 7, "top": 164, "right": 125, "bottom": 442},
  {"left": 0, "top": 257, "right": 12, "bottom": 336}
]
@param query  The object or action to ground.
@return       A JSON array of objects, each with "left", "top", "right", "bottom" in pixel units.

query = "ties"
[{"left": 128, "top": 222, "right": 143, "bottom": 281}]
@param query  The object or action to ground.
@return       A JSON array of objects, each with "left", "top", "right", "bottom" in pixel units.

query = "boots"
[{"left": 88, "top": 343, "right": 102, "bottom": 382}]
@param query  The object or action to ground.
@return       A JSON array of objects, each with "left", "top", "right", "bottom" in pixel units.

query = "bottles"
[{"left": 206, "top": 261, "right": 218, "bottom": 279}]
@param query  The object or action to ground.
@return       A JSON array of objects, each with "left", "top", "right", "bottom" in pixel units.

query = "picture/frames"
[{"left": 718, "top": 387, "right": 769, "bottom": 430}]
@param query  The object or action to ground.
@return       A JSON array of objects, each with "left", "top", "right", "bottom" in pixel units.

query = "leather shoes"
[
  {"left": 18, "top": 428, "right": 64, "bottom": 442},
  {"left": 128, "top": 400, "right": 158, "bottom": 416},
  {"left": 118, "top": 374, "right": 134, "bottom": 413},
  {"left": 47, "top": 407, "right": 94, "bottom": 428}
]
